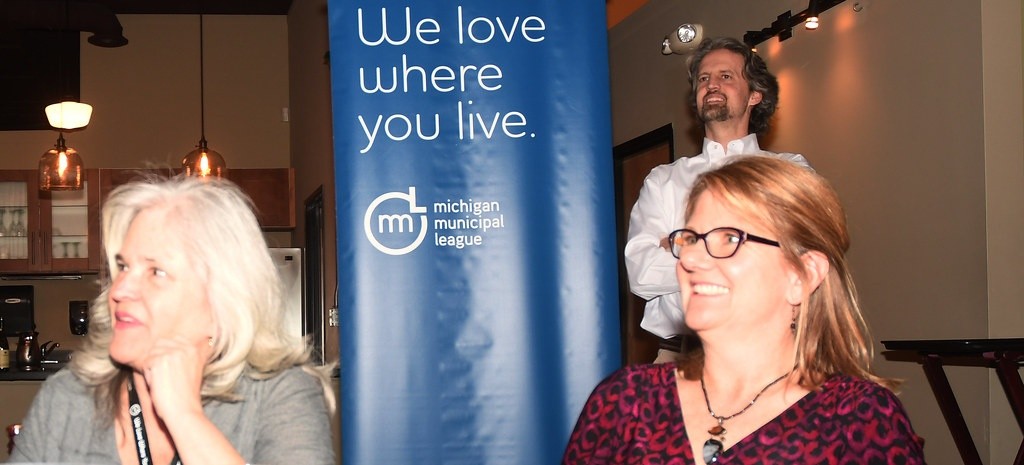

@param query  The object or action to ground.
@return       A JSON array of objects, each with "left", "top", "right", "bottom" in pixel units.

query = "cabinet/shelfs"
[{"left": 0, "top": 168, "right": 101, "bottom": 277}]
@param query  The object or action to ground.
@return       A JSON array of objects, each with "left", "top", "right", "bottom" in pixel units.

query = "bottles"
[{"left": 0, "top": 315, "right": 10, "bottom": 373}]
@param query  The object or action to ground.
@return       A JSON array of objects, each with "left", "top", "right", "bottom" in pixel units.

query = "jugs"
[{"left": 16, "top": 332, "right": 40, "bottom": 372}]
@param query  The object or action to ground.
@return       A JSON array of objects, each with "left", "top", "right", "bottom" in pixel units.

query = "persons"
[
  {"left": 624, "top": 35, "right": 818, "bottom": 365},
  {"left": 5, "top": 181, "right": 342, "bottom": 465},
  {"left": 561, "top": 155, "right": 926, "bottom": 465}
]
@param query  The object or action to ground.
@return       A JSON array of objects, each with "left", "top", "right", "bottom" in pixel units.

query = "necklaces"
[
  {"left": 696, "top": 354, "right": 800, "bottom": 465},
  {"left": 123, "top": 367, "right": 183, "bottom": 465}
]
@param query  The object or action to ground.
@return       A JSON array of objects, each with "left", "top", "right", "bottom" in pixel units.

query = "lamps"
[
  {"left": 38, "top": 131, "right": 85, "bottom": 192},
  {"left": 45, "top": 101, "right": 93, "bottom": 130},
  {"left": 661, "top": 23, "right": 703, "bottom": 55},
  {"left": 743, "top": 0, "right": 847, "bottom": 53},
  {"left": 178, "top": 0, "right": 226, "bottom": 180}
]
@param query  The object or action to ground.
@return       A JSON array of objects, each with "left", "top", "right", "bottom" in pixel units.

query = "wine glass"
[{"left": 0, "top": 209, "right": 25, "bottom": 236}]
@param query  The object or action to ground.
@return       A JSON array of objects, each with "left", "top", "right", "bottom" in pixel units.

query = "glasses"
[{"left": 669, "top": 227, "right": 781, "bottom": 260}]
